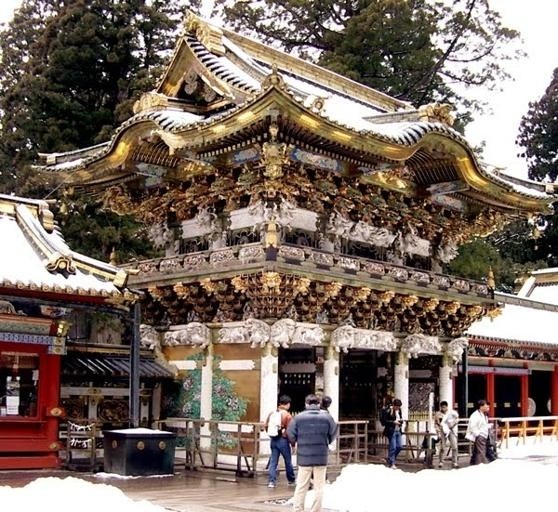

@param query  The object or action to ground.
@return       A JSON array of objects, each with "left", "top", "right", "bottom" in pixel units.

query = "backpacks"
[{"left": 266, "top": 410, "right": 284, "bottom": 438}]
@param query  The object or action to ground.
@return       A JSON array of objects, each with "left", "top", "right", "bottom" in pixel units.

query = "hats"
[{"left": 304, "top": 394, "right": 320, "bottom": 409}]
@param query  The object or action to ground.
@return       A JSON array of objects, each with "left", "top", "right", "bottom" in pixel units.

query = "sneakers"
[
  {"left": 287, "top": 481, "right": 295, "bottom": 486},
  {"left": 390, "top": 464, "right": 396, "bottom": 469},
  {"left": 452, "top": 462, "right": 458, "bottom": 468},
  {"left": 267, "top": 479, "right": 277, "bottom": 488},
  {"left": 438, "top": 461, "right": 443, "bottom": 467}
]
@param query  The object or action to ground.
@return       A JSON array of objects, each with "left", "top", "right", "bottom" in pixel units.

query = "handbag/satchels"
[{"left": 380, "top": 406, "right": 390, "bottom": 426}]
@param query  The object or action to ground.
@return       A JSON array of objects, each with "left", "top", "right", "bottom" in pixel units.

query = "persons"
[
  {"left": 265, "top": 393, "right": 340, "bottom": 512},
  {"left": 378, "top": 399, "right": 405, "bottom": 471},
  {"left": 420, "top": 399, "right": 497, "bottom": 468}
]
